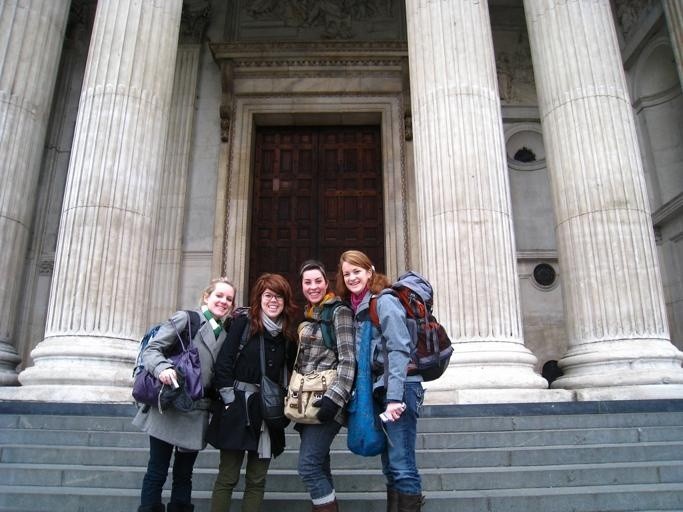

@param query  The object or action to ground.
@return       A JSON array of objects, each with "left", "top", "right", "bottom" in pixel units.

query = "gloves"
[
  {"left": 312, "top": 396, "right": 340, "bottom": 422},
  {"left": 158, "top": 368, "right": 193, "bottom": 411}
]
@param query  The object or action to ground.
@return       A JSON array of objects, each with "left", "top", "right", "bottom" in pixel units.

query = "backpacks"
[
  {"left": 133, "top": 311, "right": 200, "bottom": 404},
  {"left": 370, "top": 271, "right": 454, "bottom": 381}
]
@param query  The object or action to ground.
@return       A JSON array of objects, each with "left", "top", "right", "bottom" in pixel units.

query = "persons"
[
  {"left": 204, "top": 273, "right": 302, "bottom": 511},
  {"left": 334, "top": 249, "right": 426, "bottom": 512},
  {"left": 294, "top": 259, "right": 357, "bottom": 512},
  {"left": 130, "top": 277, "right": 239, "bottom": 511}
]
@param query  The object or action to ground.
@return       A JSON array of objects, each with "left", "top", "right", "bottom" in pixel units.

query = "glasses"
[{"left": 263, "top": 292, "right": 283, "bottom": 300}]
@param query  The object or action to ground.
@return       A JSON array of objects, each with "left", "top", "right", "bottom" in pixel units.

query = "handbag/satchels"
[
  {"left": 132, "top": 349, "right": 204, "bottom": 410},
  {"left": 347, "top": 376, "right": 385, "bottom": 456},
  {"left": 261, "top": 376, "right": 288, "bottom": 420},
  {"left": 284, "top": 369, "right": 337, "bottom": 424}
]
